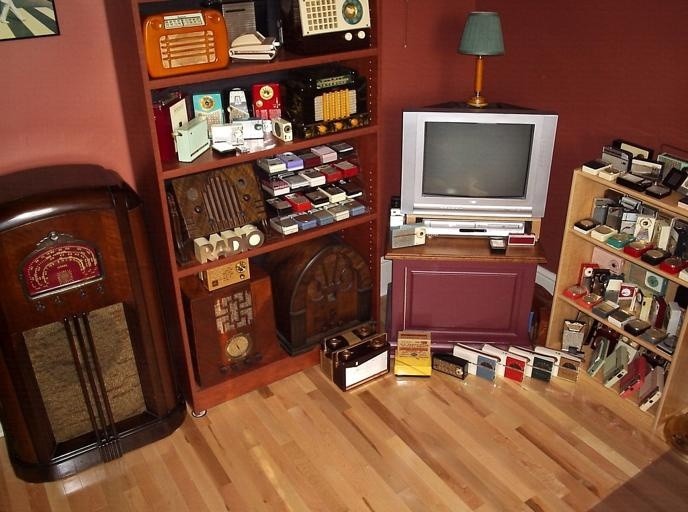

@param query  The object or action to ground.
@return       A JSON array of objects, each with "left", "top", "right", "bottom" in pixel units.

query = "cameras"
[
  {"left": 590, "top": 247, "right": 625, "bottom": 277},
  {"left": 578, "top": 263, "right": 598, "bottom": 292},
  {"left": 390, "top": 222, "right": 426, "bottom": 249},
  {"left": 590, "top": 269, "right": 610, "bottom": 296}
]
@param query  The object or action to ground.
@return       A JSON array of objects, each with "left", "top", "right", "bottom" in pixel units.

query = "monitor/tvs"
[{"left": 400, "top": 101, "right": 559, "bottom": 218}]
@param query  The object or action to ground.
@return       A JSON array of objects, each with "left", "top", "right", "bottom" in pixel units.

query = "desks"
[{"left": 376, "top": 240, "right": 547, "bottom": 358}]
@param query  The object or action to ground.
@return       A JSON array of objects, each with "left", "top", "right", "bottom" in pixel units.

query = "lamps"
[{"left": 457, "top": 10, "right": 508, "bottom": 109}]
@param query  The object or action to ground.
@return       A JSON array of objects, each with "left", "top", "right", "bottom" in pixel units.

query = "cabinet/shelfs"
[
  {"left": 542, "top": 163, "right": 688, "bottom": 435},
  {"left": 105, "top": 1, "right": 388, "bottom": 418}
]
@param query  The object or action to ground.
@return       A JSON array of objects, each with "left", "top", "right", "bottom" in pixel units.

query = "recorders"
[
  {"left": 142, "top": 9, "right": 229, "bottom": 79},
  {"left": 333, "top": 332, "right": 390, "bottom": 392},
  {"left": 283, "top": 0, "right": 372, "bottom": 55},
  {"left": 320, "top": 323, "right": 379, "bottom": 381}
]
